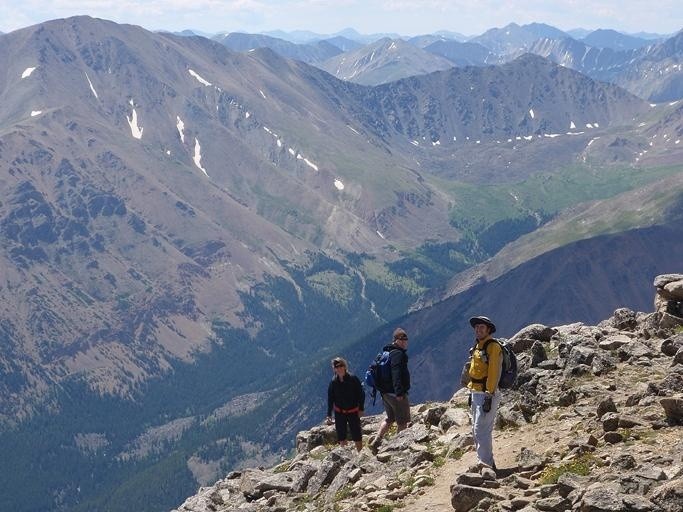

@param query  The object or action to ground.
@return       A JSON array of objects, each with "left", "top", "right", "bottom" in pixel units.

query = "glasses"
[{"left": 394, "top": 335, "right": 407, "bottom": 340}]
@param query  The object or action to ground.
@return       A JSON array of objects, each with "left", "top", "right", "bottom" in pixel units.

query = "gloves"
[{"left": 483, "top": 399, "right": 491, "bottom": 412}]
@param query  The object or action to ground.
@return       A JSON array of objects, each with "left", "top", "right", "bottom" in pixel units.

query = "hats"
[
  {"left": 470, "top": 316, "right": 496, "bottom": 333},
  {"left": 392, "top": 327, "right": 407, "bottom": 342}
]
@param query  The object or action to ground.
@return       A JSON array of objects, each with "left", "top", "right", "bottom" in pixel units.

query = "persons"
[
  {"left": 325, "top": 356, "right": 365, "bottom": 453},
  {"left": 366, "top": 328, "right": 411, "bottom": 453},
  {"left": 462, "top": 315, "right": 517, "bottom": 468}
]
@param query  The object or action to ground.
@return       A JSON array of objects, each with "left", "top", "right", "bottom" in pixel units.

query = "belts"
[{"left": 333, "top": 404, "right": 359, "bottom": 414}]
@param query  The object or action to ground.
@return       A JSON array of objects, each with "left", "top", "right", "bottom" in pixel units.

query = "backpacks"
[
  {"left": 469, "top": 338, "right": 517, "bottom": 398},
  {"left": 364, "top": 347, "right": 405, "bottom": 407}
]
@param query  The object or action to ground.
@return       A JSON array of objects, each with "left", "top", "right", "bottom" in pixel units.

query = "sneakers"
[
  {"left": 466, "top": 463, "right": 497, "bottom": 474},
  {"left": 367, "top": 441, "right": 379, "bottom": 456}
]
